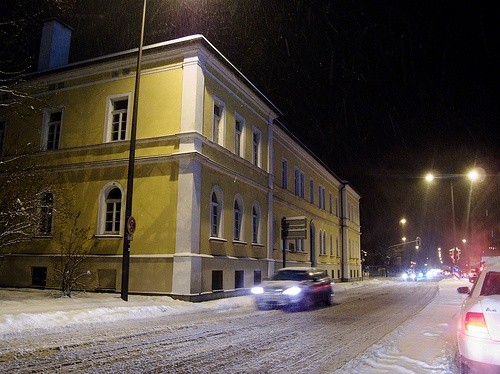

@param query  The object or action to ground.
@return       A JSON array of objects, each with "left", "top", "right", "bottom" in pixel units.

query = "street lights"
[{"left": 420, "top": 168, "right": 486, "bottom": 272}]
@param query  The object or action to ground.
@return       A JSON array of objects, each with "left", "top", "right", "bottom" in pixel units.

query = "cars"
[
  {"left": 395, "top": 266, "right": 481, "bottom": 285},
  {"left": 453, "top": 264, "right": 500, "bottom": 374},
  {"left": 252, "top": 264, "right": 336, "bottom": 311}
]
